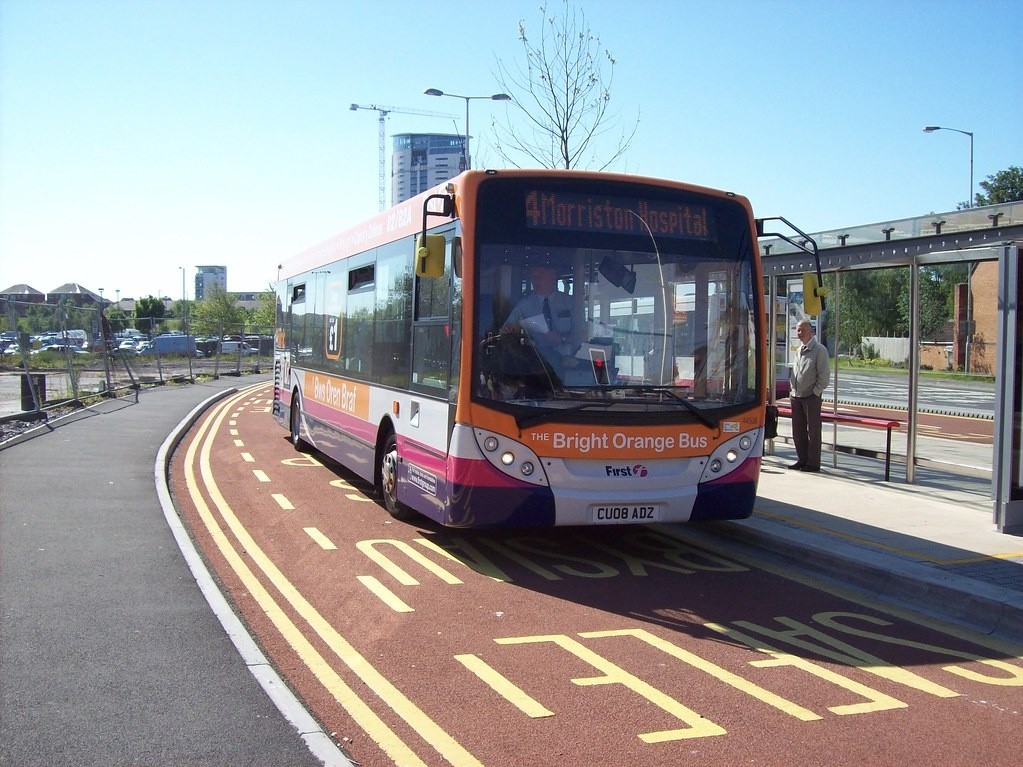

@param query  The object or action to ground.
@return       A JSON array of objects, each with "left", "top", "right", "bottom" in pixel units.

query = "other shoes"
[
  {"left": 788, "top": 463, "right": 803, "bottom": 469},
  {"left": 800, "top": 465, "right": 819, "bottom": 471}
]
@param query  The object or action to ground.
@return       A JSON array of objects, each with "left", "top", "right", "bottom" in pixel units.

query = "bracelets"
[{"left": 562, "top": 335, "right": 565, "bottom": 343}]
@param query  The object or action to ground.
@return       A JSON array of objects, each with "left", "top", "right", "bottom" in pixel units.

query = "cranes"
[{"left": 350, "top": 102, "right": 462, "bottom": 213}]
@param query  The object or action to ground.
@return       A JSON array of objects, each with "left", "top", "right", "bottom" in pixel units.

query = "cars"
[{"left": 0, "top": 330, "right": 270, "bottom": 357}]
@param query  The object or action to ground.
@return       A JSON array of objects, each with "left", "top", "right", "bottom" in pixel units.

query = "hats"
[{"left": 533, "top": 258, "right": 565, "bottom": 277}]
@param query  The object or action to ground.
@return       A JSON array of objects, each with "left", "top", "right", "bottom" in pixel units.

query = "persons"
[
  {"left": 788, "top": 319, "right": 830, "bottom": 472},
  {"left": 500, "top": 262, "right": 587, "bottom": 362}
]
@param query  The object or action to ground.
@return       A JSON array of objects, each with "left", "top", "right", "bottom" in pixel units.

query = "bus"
[{"left": 269, "top": 168, "right": 830, "bottom": 530}]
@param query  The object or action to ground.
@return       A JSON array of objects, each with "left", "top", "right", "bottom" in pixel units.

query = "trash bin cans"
[{"left": 20, "top": 374, "right": 47, "bottom": 411}]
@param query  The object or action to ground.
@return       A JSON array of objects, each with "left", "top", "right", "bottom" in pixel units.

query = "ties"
[{"left": 543, "top": 297, "right": 553, "bottom": 332}]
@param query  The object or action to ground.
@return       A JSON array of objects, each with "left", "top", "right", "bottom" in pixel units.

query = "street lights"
[
  {"left": 98, "top": 288, "right": 104, "bottom": 313},
  {"left": 115, "top": 289, "right": 121, "bottom": 308},
  {"left": 425, "top": 89, "right": 511, "bottom": 173},
  {"left": 922, "top": 125, "right": 973, "bottom": 209},
  {"left": 179, "top": 266, "right": 186, "bottom": 335}
]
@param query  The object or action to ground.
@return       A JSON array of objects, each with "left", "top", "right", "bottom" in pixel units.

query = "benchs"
[{"left": 776, "top": 407, "right": 900, "bottom": 481}]
